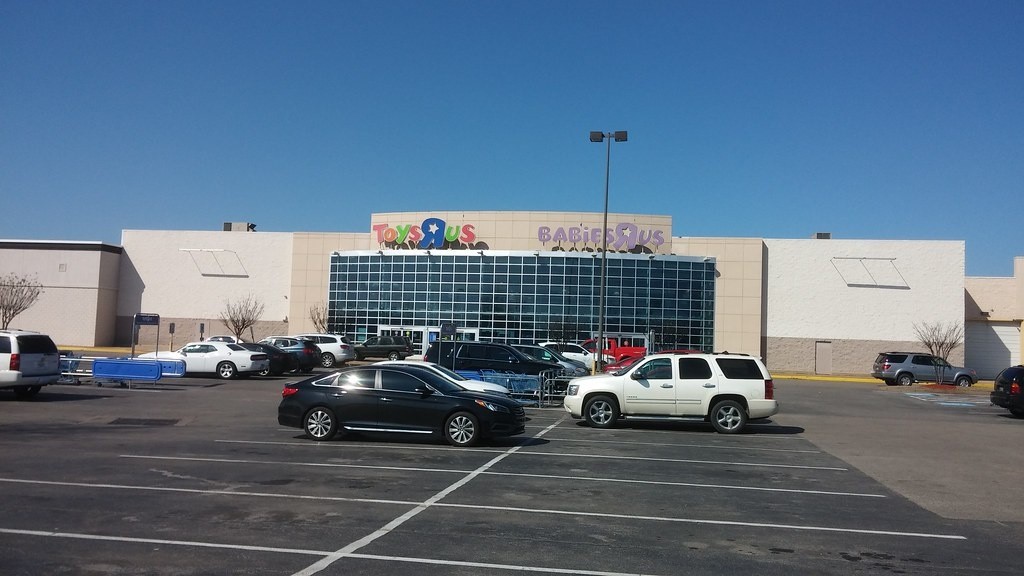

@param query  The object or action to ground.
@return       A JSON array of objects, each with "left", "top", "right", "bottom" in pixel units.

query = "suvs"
[
  {"left": 989, "top": 365, "right": 1024, "bottom": 418},
  {"left": 256, "top": 336, "right": 322, "bottom": 374},
  {"left": 294, "top": 334, "right": 354, "bottom": 368},
  {"left": 871, "top": 351, "right": 978, "bottom": 388},
  {"left": 508, "top": 343, "right": 589, "bottom": 380},
  {"left": 0, "top": 329, "right": 61, "bottom": 396},
  {"left": 539, "top": 342, "right": 613, "bottom": 372},
  {"left": 564, "top": 350, "right": 779, "bottom": 434},
  {"left": 354, "top": 335, "right": 414, "bottom": 361},
  {"left": 424, "top": 340, "right": 568, "bottom": 400}
]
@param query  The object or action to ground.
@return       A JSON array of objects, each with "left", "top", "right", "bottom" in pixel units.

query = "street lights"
[{"left": 590, "top": 131, "right": 627, "bottom": 370}]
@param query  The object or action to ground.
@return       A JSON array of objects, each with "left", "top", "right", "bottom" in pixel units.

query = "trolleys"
[
  {"left": 481, "top": 369, "right": 506, "bottom": 387},
  {"left": 506, "top": 371, "right": 542, "bottom": 408}
]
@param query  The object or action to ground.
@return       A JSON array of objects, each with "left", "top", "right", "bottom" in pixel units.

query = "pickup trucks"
[{"left": 579, "top": 339, "right": 646, "bottom": 362}]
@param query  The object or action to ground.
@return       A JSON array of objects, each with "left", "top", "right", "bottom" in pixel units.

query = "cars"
[
  {"left": 138, "top": 341, "right": 269, "bottom": 379},
  {"left": 604, "top": 356, "right": 643, "bottom": 373},
  {"left": 277, "top": 365, "right": 526, "bottom": 447},
  {"left": 372, "top": 361, "right": 513, "bottom": 400},
  {"left": 204, "top": 335, "right": 245, "bottom": 343},
  {"left": 233, "top": 343, "right": 299, "bottom": 377}
]
